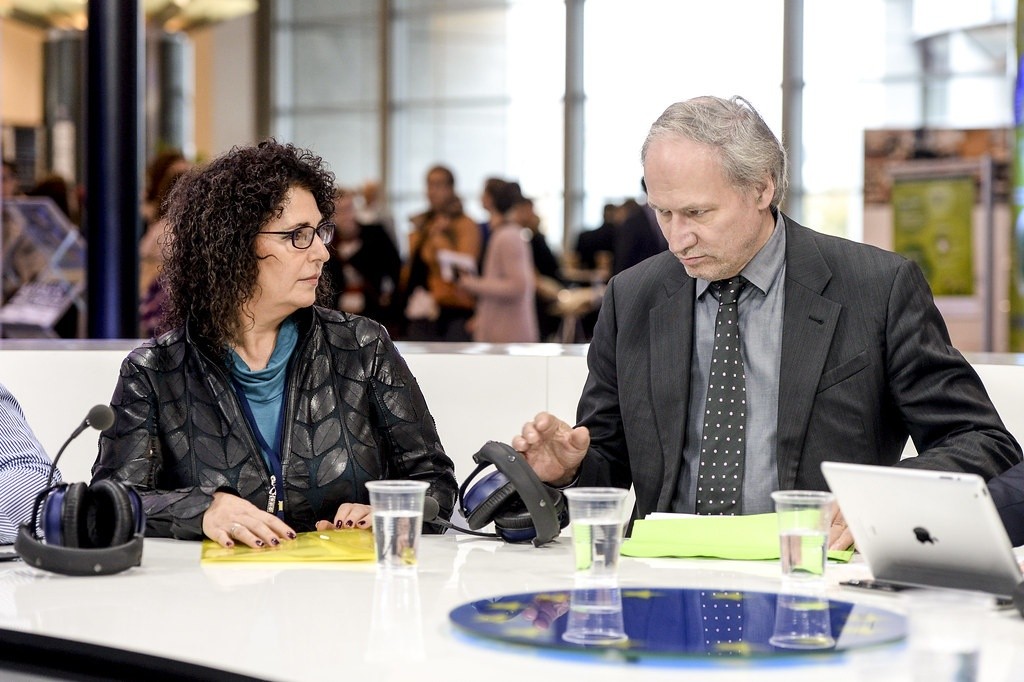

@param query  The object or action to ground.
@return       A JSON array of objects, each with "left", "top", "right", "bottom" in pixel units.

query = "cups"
[
  {"left": 561, "top": 574, "right": 628, "bottom": 646},
  {"left": 770, "top": 489, "right": 834, "bottom": 577},
  {"left": 364, "top": 480, "right": 430, "bottom": 566},
  {"left": 562, "top": 487, "right": 629, "bottom": 574},
  {"left": 768, "top": 577, "right": 836, "bottom": 650}
]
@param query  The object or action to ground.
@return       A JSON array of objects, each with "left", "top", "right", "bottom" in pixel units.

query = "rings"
[{"left": 231, "top": 524, "right": 241, "bottom": 537}]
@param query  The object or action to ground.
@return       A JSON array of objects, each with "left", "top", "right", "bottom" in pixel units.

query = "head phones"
[
  {"left": 13, "top": 481, "right": 147, "bottom": 576},
  {"left": 458, "top": 441, "right": 570, "bottom": 548}
]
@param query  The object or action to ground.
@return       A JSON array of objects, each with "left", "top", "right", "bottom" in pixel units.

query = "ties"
[{"left": 694, "top": 274, "right": 748, "bottom": 515}]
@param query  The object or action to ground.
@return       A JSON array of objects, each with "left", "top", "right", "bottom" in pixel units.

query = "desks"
[{"left": 0, "top": 538, "right": 1024, "bottom": 682}]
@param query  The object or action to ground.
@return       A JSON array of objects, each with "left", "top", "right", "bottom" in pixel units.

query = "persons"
[
  {"left": 511, "top": 94, "right": 1024, "bottom": 554},
  {"left": 317, "top": 165, "right": 669, "bottom": 344},
  {"left": 0, "top": 160, "right": 88, "bottom": 339},
  {"left": 89, "top": 140, "right": 459, "bottom": 553},
  {"left": 135, "top": 150, "right": 195, "bottom": 338},
  {"left": 0, "top": 381, "right": 62, "bottom": 547}
]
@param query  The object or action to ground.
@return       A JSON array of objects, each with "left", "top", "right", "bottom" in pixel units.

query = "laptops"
[{"left": 818, "top": 461, "right": 1024, "bottom": 597}]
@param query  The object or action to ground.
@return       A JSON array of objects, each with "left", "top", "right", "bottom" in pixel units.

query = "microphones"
[
  {"left": 421, "top": 495, "right": 496, "bottom": 538},
  {"left": 49, "top": 405, "right": 115, "bottom": 486}
]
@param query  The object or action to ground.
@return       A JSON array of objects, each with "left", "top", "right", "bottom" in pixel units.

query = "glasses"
[{"left": 257, "top": 221, "right": 335, "bottom": 250}]
